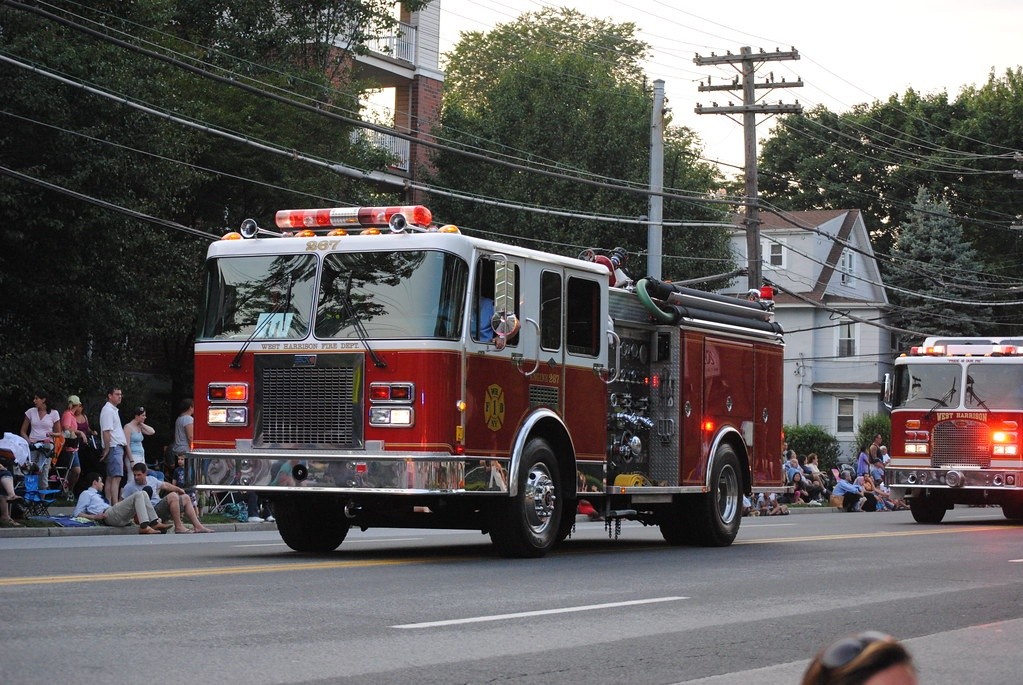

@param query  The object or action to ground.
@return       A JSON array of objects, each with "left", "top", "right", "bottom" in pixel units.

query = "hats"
[
  {"left": 179, "top": 399, "right": 192, "bottom": 412},
  {"left": 67, "top": 395, "right": 81, "bottom": 405},
  {"left": 134, "top": 406, "right": 144, "bottom": 416}
]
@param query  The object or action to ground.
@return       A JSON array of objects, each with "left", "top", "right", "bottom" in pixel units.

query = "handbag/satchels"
[{"left": 830, "top": 495, "right": 844, "bottom": 508}]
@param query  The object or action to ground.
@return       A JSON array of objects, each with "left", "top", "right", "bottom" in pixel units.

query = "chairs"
[
  {"left": 207, "top": 489, "right": 245, "bottom": 515},
  {"left": 22, "top": 475, "right": 61, "bottom": 517}
]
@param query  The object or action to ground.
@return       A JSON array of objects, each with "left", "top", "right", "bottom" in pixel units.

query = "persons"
[
  {"left": 72, "top": 472, "right": 173, "bottom": 533},
  {"left": 800, "top": 629, "right": 918, "bottom": 685},
  {"left": 577, "top": 498, "right": 606, "bottom": 521},
  {"left": 739, "top": 434, "right": 910, "bottom": 516},
  {"left": 0, "top": 463, "right": 24, "bottom": 528},
  {"left": 171, "top": 450, "right": 201, "bottom": 521},
  {"left": 424, "top": 269, "right": 521, "bottom": 350},
  {"left": 124, "top": 406, "right": 155, "bottom": 483},
  {"left": 99, "top": 383, "right": 127, "bottom": 507},
  {"left": 172, "top": 398, "right": 193, "bottom": 470},
  {"left": 60, "top": 395, "right": 81, "bottom": 490},
  {"left": 220, "top": 493, "right": 276, "bottom": 522},
  {"left": 118, "top": 462, "right": 215, "bottom": 533},
  {"left": 20, "top": 388, "right": 62, "bottom": 507},
  {"left": 74, "top": 398, "right": 98, "bottom": 470}
]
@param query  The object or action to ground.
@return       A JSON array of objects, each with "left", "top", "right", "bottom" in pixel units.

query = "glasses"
[{"left": 811, "top": 630, "right": 894, "bottom": 684}]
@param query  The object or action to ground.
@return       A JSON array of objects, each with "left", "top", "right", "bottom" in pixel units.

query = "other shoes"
[
  {"left": 0, "top": 517, "right": 20, "bottom": 528},
  {"left": 6, "top": 495, "right": 24, "bottom": 504},
  {"left": 266, "top": 516, "right": 275, "bottom": 522},
  {"left": 247, "top": 517, "right": 264, "bottom": 523},
  {"left": 151, "top": 522, "right": 173, "bottom": 530},
  {"left": 139, "top": 526, "right": 161, "bottom": 534},
  {"left": 809, "top": 500, "right": 822, "bottom": 506},
  {"left": 854, "top": 505, "right": 912, "bottom": 512}
]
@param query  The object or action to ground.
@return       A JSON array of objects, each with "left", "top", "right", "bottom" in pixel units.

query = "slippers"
[
  {"left": 175, "top": 528, "right": 194, "bottom": 534},
  {"left": 193, "top": 527, "right": 215, "bottom": 533}
]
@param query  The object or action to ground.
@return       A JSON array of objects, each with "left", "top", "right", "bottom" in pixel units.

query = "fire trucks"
[
  {"left": 879, "top": 336, "right": 1023, "bottom": 523},
  {"left": 183, "top": 204, "right": 794, "bottom": 560}
]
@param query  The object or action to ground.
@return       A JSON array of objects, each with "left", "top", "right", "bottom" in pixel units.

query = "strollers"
[
  {"left": 10, "top": 439, "right": 55, "bottom": 495},
  {"left": 48, "top": 429, "right": 89, "bottom": 502}
]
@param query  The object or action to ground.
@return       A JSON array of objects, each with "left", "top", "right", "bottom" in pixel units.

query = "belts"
[{"left": 103, "top": 507, "right": 110, "bottom": 525}]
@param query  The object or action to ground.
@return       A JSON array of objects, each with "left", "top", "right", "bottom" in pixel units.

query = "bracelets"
[
  {"left": 130, "top": 461, "right": 134, "bottom": 463},
  {"left": 499, "top": 336, "right": 504, "bottom": 340}
]
